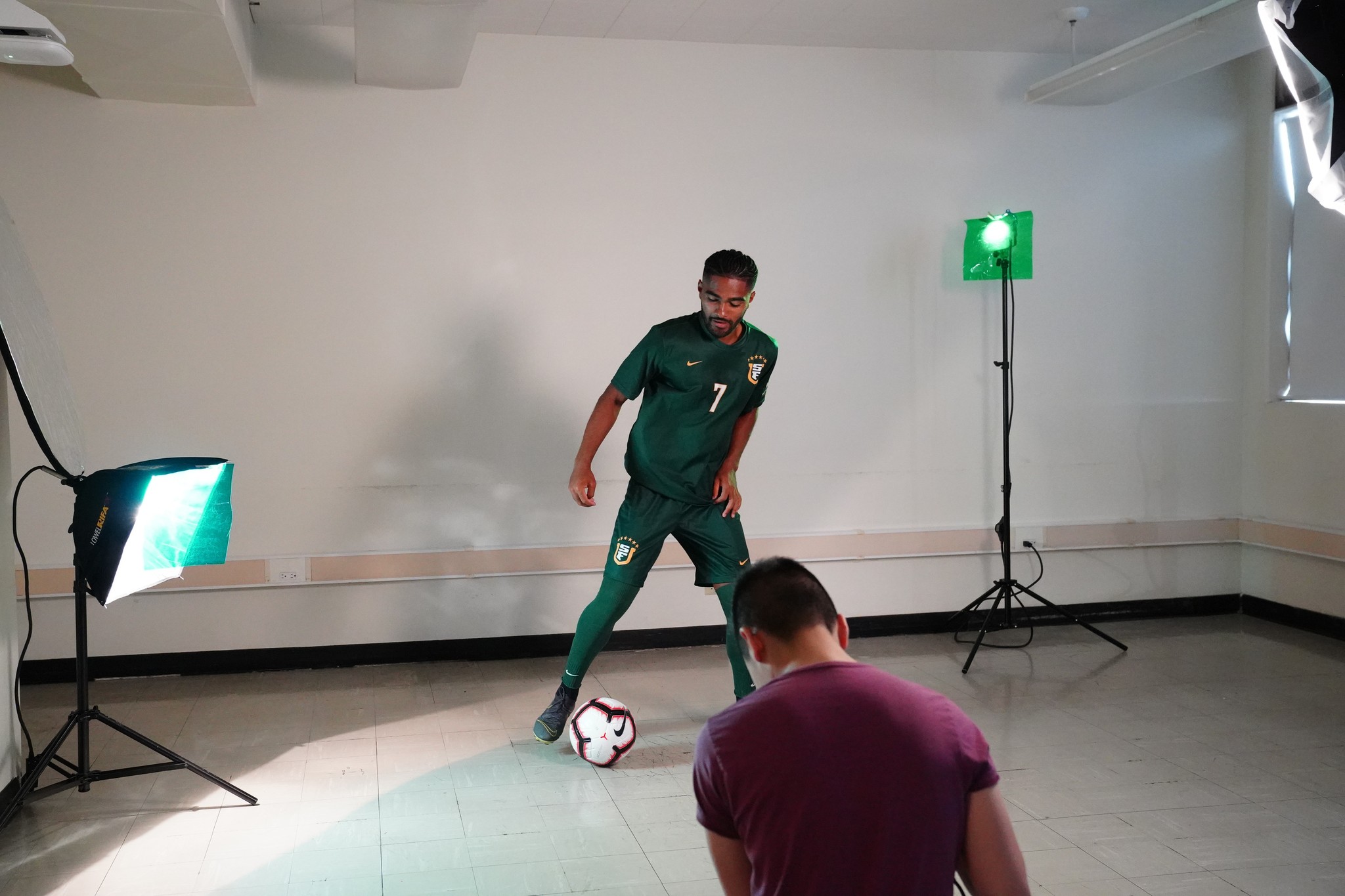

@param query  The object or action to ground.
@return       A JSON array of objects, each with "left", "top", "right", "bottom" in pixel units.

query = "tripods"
[
  {"left": 0, "top": 525, "right": 258, "bottom": 828},
  {"left": 945, "top": 261, "right": 1128, "bottom": 678}
]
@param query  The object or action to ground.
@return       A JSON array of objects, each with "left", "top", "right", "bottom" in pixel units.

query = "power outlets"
[
  {"left": 1016, "top": 527, "right": 1042, "bottom": 550},
  {"left": 269, "top": 559, "right": 306, "bottom": 582}
]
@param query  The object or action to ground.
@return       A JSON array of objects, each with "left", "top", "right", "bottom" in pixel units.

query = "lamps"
[{"left": 0, "top": 0, "right": 73, "bottom": 66}]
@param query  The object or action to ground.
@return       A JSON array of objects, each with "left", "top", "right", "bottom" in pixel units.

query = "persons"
[
  {"left": 534, "top": 248, "right": 777, "bottom": 744},
  {"left": 691, "top": 555, "right": 1031, "bottom": 895}
]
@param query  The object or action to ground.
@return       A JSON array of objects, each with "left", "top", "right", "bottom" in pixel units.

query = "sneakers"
[{"left": 533, "top": 682, "right": 579, "bottom": 745}]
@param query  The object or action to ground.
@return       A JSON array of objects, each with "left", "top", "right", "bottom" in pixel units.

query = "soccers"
[{"left": 569, "top": 697, "right": 637, "bottom": 767}]
